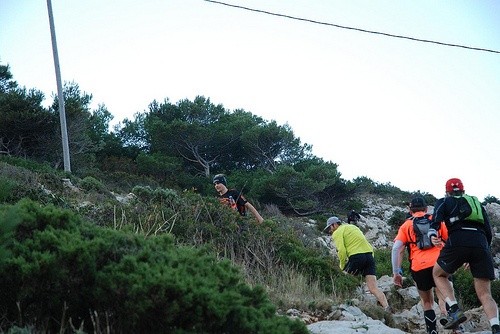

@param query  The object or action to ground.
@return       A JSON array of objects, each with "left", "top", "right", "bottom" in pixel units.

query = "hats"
[
  {"left": 409, "top": 197, "right": 427, "bottom": 208},
  {"left": 445, "top": 178, "right": 464, "bottom": 193},
  {"left": 323, "top": 217, "right": 341, "bottom": 231},
  {"left": 213, "top": 173, "right": 227, "bottom": 186}
]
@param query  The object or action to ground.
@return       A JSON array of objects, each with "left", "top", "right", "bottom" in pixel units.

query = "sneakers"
[
  {"left": 440, "top": 312, "right": 457, "bottom": 327},
  {"left": 492, "top": 324, "right": 500, "bottom": 334},
  {"left": 443, "top": 309, "right": 466, "bottom": 326}
]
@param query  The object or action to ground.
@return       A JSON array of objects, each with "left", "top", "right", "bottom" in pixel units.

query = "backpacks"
[
  {"left": 407, "top": 213, "right": 435, "bottom": 250},
  {"left": 453, "top": 194, "right": 484, "bottom": 225}
]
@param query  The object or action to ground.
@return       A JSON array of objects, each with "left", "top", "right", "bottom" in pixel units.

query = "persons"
[
  {"left": 323, "top": 216, "right": 391, "bottom": 313},
  {"left": 213, "top": 173, "right": 264, "bottom": 225},
  {"left": 427, "top": 178, "right": 500, "bottom": 334},
  {"left": 391, "top": 196, "right": 448, "bottom": 334},
  {"left": 347, "top": 209, "right": 360, "bottom": 224}
]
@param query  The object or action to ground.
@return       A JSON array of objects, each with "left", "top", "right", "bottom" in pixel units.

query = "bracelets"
[{"left": 428, "top": 229, "right": 438, "bottom": 238}]
